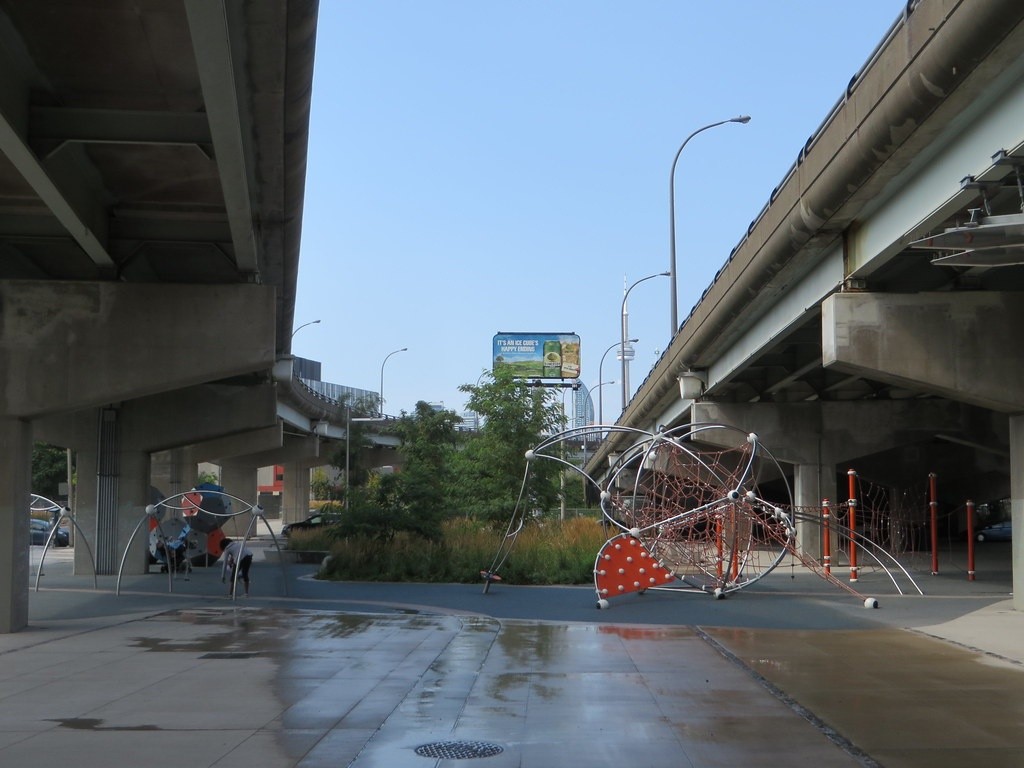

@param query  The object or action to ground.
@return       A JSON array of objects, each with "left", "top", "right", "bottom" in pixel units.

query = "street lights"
[
  {"left": 620, "top": 272, "right": 671, "bottom": 411},
  {"left": 599, "top": 339, "right": 639, "bottom": 445},
  {"left": 668, "top": 115, "right": 751, "bottom": 340},
  {"left": 583, "top": 380, "right": 615, "bottom": 465},
  {"left": 380, "top": 348, "right": 408, "bottom": 415}
]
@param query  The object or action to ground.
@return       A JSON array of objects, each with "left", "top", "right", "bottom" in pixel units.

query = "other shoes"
[
  {"left": 241, "top": 593, "right": 249, "bottom": 598},
  {"left": 223, "top": 593, "right": 233, "bottom": 599}
]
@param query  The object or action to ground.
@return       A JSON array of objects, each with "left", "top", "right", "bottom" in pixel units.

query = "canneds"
[{"left": 542, "top": 340, "right": 579, "bottom": 378}]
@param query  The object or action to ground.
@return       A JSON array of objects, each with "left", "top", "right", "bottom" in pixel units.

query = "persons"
[{"left": 219, "top": 538, "right": 253, "bottom": 600}]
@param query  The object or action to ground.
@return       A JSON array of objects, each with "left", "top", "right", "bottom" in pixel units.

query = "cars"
[
  {"left": 30, "top": 519, "right": 70, "bottom": 548},
  {"left": 974, "top": 521, "right": 1012, "bottom": 544},
  {"left": 281, "top": 514, "right": 337, "bottom": 539}
]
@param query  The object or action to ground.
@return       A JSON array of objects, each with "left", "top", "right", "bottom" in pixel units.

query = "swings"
[
  {"left": 214, "top": 513, "right": 249, "bottom": 582},
  {"left": 28, "top": 508, "right": 49, "bottom": 576},
  {"left": 169, "top": 507, "right": 195, "bottom": 582}
]
